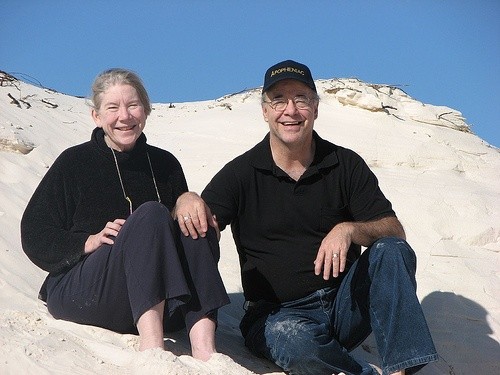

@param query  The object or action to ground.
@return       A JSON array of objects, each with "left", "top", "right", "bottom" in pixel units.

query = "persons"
[
  {"left": 174, "top": 60, "right": 439, "bottom": 375},
  {"left": 20, "top": 67, "right": 255, "bottom": 375}
]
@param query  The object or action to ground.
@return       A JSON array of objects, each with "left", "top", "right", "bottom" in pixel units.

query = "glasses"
[{"left": 264, "top": 93, "right": 316, "bottom": 111}]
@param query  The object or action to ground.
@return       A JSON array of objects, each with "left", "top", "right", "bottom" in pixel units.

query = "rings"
[
  {"left": 183, "top": 217, "right": 190, "bottom": 221},
  {"left": 333, "top": 254, "right": 338, "bottom": 257}
]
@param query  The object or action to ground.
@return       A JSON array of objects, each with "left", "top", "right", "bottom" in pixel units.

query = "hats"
[{"left": 263, "top": 60, "right": 316, "bottom": 93}]
